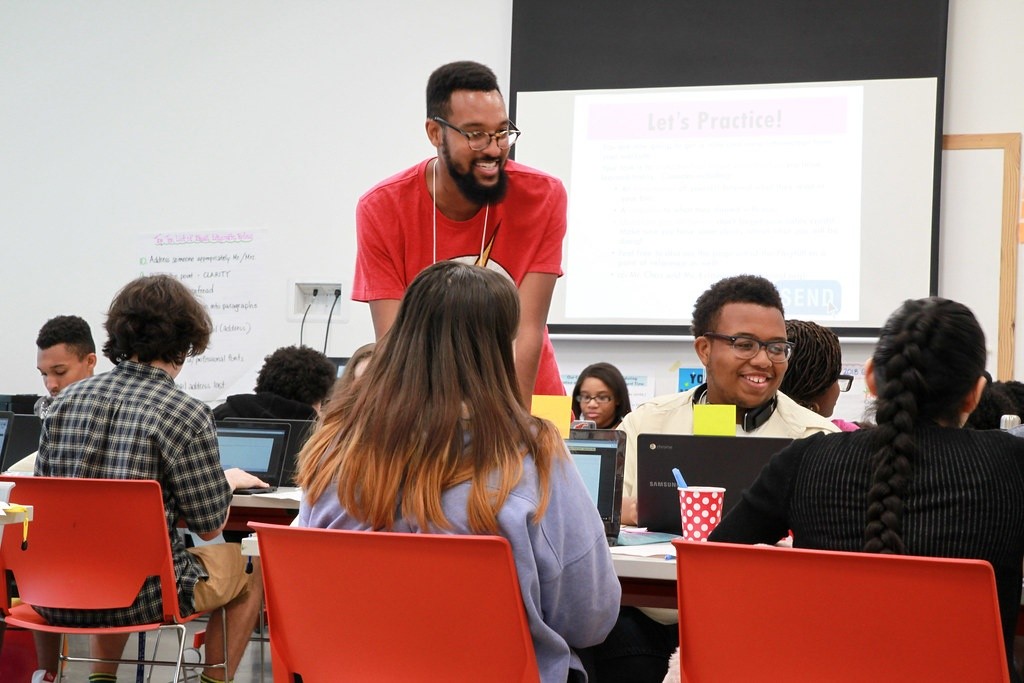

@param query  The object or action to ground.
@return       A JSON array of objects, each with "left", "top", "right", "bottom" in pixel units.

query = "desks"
[
  {"left": 175, "top": 486, "right": 303, "bottom": 532},
  {"left": 609, "top": 524, "right": 794, "bottom": 609}
]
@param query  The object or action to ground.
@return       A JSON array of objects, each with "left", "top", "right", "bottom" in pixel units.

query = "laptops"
[
  {"left": 559, "top": 426, "right": 626, "bottom": 547},
  {"left": 637, "top": 434, "right": 794, "bottom": 537},
  {"left": 0, "top": 411, "right": 44, "bottom": 479},
  {"left": 214, "top": 417, "right": 318, "bottom": 495}
]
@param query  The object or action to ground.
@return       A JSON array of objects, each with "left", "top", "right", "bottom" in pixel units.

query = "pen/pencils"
[{"left": 665, "top": 554, "right": 677, "bottom": 560}]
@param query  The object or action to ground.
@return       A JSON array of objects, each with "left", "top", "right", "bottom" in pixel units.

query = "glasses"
[
  {"left": 434, "top": 116, "right": 522, "bottom": 152},
  {"left": 575, "top": 395, "right": 615, "bottom": 404},
  {"left": 703, "top": 331, "right": 796, "bottom": 363},
  {"left": 836, "top": 375, "right": 854, "bottom": 392}
]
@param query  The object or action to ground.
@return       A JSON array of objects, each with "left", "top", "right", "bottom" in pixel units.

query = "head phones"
[{"left": 691, "top": 382, "right": 778, "bottom": 432}]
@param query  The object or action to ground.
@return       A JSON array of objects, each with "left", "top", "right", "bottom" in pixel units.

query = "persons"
[
  {"left": 29, "top": 273, "right": 264, "bottom": 682},
  {"left": 778, "top": 320, "right": 861, "bottom": 432},
  {"left": 963, "top": 380, "right": 1024, "bottom": 432},
  {"left": 252, "top": 345, "right": 338, "bottom": 419},
  {"left": 296, "top": 261, "right": 623, "bottom": 683},
  {"left": 30, "top": 314, "right": 97, "bottom": 682},
  {"left": 614, "top": 274, "right": 844, "bottom": 526},
  {"left": 707, "top": 296, "right": 1024, "bottom": 683},
  {"left": 572, "top": 362, "right": 632, "bottom": 430},
  {"left": 350, "top": 61, "right": 576, "bottom": 395}
]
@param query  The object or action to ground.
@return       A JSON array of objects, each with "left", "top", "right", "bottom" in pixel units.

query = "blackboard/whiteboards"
[{"left": 938, "top": 133, "right": 1022, "bottom": 382}]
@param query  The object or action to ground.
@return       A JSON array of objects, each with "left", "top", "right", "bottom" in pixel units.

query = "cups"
[{"left": 677, "top": 486, "right": 726, "bottom": 542}]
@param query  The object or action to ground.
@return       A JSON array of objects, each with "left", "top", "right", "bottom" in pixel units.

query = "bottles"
[{"left": 1000, "top": 414, "right": 1021, "bottom": 433}]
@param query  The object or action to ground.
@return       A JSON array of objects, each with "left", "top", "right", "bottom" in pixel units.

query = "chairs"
[
  {"left": 247, "top": 520, "right": 542, "bottom": 683},
  {"left": 669, "top": 539, "right": 1011, "bottom": 683},
  {"left": 0, "top": 477, "right": 230, "bottom": 683}
]
